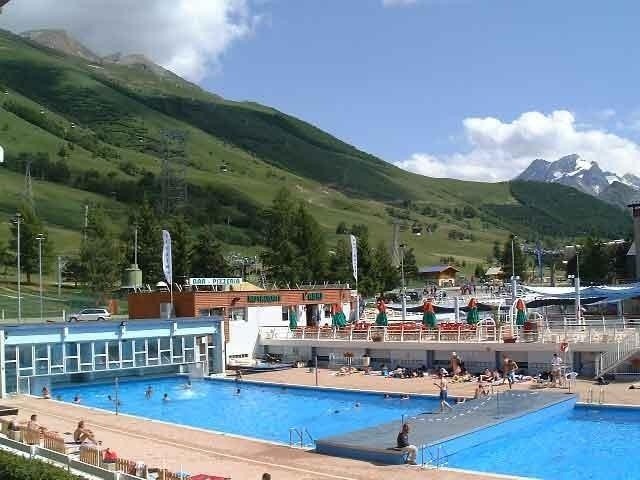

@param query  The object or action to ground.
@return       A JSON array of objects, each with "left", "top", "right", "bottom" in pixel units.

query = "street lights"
[
  {"left": 572, "top": 242, "right": 583, "bottom": 332},
  {"left": 35, "top": 231, "right": 48, "bottom": 321},
  {"left": 397, "top": 241, "right": 408, "bottom": 325},
  {"left": 11, "top": 212, "right": 27, "bottom": 326},
  {"left": 508, "top": 231, "right": 518, "bottom": 333}
]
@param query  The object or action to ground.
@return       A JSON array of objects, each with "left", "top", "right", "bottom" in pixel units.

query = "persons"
[
  {"left": 237, "top": 388, "right": 241, "bottom": 394},
  {"left": 185, "top": 380, "right": 192, "bottom": 392},
  {"left": 72, "top": 396, "right": 80, "bottom": 404},
  {"left": 450, "top": 351, "right": 462, "bottom": 376},
  {"left": 397, "top": 423, "right": 417, "bottom": 465},
  {"left": 400, "top": 395, "right": 408, "bottom": 401},
  {"left": 263, "top": 473, "right": 271, "bottom": 480},
  {"left": 433, "top": 372, "right": 452, "bottom": 412},
  {"left": 551, "top": 353, "right": 563, "bottom": 386},
  {"left": 356, "top": 403, "right": 360, "bottom": 407},
  {"left": 162, "top": 393, "right": 169, "bottom": 400},
  {"left": 74, "top": 420, "right": 97, "bottom": 444},
  {"left": 384, "top": 393, "right": 390, "bottom": 398},
  {"left": 41, "top": 385, "right": 49, "bottom": 395},
  {"left": 484, "top": 353, "right": 518, "bottom": 389},
  {"left": 108, "top": 395, "right": 120, "bottom": 404},
  {"left": 430, "top": 286, "right": 437, "bottom": 294},
  {"left": 145, "top": 386, "right": 153, "bottom": 399},
  {"left": 26, "top": 414, "right": 48, "bottom": 434}
]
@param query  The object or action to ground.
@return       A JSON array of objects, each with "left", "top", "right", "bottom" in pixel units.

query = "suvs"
[{"left": 65, "top": 306, "right": 114, "bottom": 322}]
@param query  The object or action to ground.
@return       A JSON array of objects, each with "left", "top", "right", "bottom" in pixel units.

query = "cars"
[{"left": 377, "top": 291, "right": 420, "bottom": 304}]
